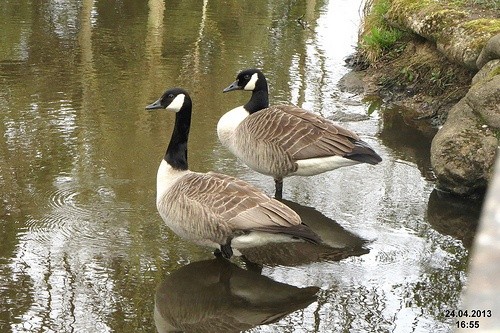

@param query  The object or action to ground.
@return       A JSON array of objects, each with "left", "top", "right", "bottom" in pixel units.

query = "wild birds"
[
  {"left": 146, "top": 88, "right": 325, "bottom": 262},
  {"left": 216, "top": 67, "right": 383, "bottom": 192},
  {"left": 236, "top": 193, "right": 372, "bottom": 273},
  {"left": 150, "top": 259, "right": 323, "bottom": 333}
]
